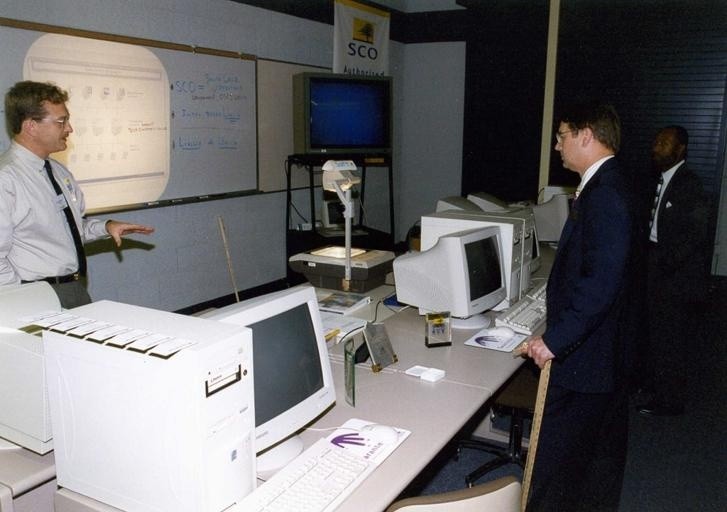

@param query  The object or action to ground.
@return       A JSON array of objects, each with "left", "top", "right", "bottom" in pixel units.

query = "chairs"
[
  {"left": 384, "top": 472, "right": 523, "bottom": 512},
  {"left": 448, "top": 366, "right": 540, "bottom": 490}
]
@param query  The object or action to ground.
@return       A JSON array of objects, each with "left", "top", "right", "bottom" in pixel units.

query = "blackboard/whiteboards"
[
  {"left": 256, "top": 55, "right": 332, "bottom": 194},
  {"left": 0, "top": 17, "right": 258, "bottom": 219}
]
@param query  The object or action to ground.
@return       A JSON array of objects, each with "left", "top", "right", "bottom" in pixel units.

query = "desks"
[
  {"left": 0, "top": 244, "right": 557, "bottom": 512},
  {"left": 283, "top": 153, "right": 397, "bottom": 286}
]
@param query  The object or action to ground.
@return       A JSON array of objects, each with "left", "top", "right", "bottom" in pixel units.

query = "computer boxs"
[
  {"left": 447, "top": 207, "right": 534, "bottom": 299},
  {"left": 420, "top": 212, "right": 524, "bottom": 310},
  {"left": 39, "top": 298, "right": 255, "bottom": 512}
]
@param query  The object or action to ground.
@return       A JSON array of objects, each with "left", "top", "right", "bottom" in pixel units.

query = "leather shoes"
[{"left": 639, "top": 404, "right": 682, "bottom": 416}]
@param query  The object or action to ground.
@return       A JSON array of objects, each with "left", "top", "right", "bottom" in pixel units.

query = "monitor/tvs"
[
  {"left": 436, "top": 196, "right": 486, "bottom": 213},
  {"left": 530, "top": 225, "right": 543, "bottom": 277},
  {"left": 393, "top": 225, "right": 508, "bottom": 330},
  {"left": 292, "top": 71, "right": 395, "bottom": 161},
  {"left": 533, "top": 194, "right": 575, "bottom": 250},
  {"left": 468, "top": 191, "right": 509, "bottom": 211},
  {"left": 200, "top": 281, "right": 338, "bottom": 478}
]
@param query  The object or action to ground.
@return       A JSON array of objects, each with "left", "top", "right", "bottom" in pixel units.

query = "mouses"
[
  {"left": 493, "top": 326, "right": 515, "bottom": 338},
  {"left": 359, "top": 422, "right": 402, "bottom": 447}
]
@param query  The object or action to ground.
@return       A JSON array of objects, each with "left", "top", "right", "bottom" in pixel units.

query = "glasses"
[
  {"left": 32, "top": 117, "right": 67, "bottom": 128},
  {"left": 556, "top": 125, "right": 593, "bottom": 144}
]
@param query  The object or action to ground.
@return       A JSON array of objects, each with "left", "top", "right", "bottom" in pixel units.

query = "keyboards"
[
  {"left": 229, "top": 438, "right": 375, "bottom": 512},
  {"left": 494, "top": 278, "right": 554, "bottom": 337}
]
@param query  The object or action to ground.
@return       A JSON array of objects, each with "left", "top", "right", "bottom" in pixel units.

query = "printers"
[{"left": 0, "top": 254, "right": 66, "bottom": 457}]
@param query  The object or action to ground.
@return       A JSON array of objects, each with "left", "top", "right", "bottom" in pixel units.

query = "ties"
[
  {"left": 44, "top": 160, "right": 86, "bottom": 277},
  {"left": 649, "top": 176, "right": 663, "bottom": 228}
]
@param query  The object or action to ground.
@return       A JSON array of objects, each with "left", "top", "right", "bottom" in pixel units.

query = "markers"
[
  {"left": 144, "top": 202, "right": 160, "bottom": 205},
  {"left": 168, "top": 199, "right": 183, "bottom": 203},
  {"left": 196, "top": 196, "right": 209, "bottom": 200}
]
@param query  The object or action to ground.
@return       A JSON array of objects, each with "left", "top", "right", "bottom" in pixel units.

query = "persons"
[
  {"left": 522, "top": 103, "right": 650, "bottom": 512},
  {"left": 0, "top": 81, "right": 155, "bottom": 309},
  {"left": 636, "top": 124, "right": 708, "bottom": 415}
]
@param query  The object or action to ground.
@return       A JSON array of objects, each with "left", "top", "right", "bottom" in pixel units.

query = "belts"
[{"left": 21, "top": 272, "right": 80, "bottom": 284}]
